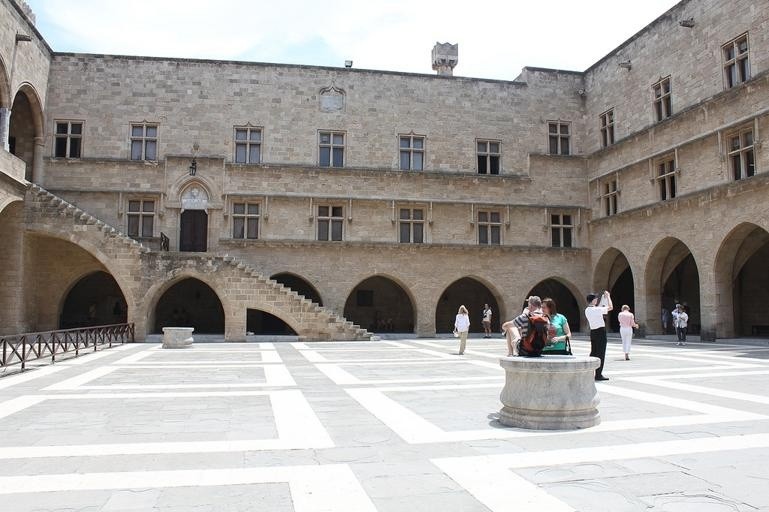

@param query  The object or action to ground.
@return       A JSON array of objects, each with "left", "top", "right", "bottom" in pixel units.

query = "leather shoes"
[{"left": 595, "top": 376, "right": 609, "bottom": 380}]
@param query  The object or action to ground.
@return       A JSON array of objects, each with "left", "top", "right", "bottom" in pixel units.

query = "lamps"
[
  {"left": 345, "top": 60, "right": 352, "bottom": 67},
  {"left": 189, "top": 160, "right": 196, "bottom": 176}
]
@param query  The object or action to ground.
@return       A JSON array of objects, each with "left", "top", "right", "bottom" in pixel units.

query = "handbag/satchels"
[
  {"left": 565, "top": 336, "right": 572, "bottom": 354},
  {"left": 453, "top": 326, "right": 459, "bottom": 338}
]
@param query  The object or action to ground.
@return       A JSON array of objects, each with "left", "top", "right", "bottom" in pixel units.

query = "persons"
[
  {"left": 660, "top": 297, "right": 690, "bottom": 346},
  {"left": 540, "top": 297, "right": 572, "bottom": 354},
  {"left": 522, "top": 295, "right": 534, "bottom": 314},
  {"left": 454, "top": 304, "right": 470, "bottom": 355},
  {"left": 584, "top": 290, "right": 614, "bottom": 381},
  {"left": 616, "top": 304, "right": 639, "bottom": 361},
  {"left": 501, "top": 295, "right": 549, "bottom": 358},
  {"left": 482, "top": 303, "right": 493, "bottom": 338}
]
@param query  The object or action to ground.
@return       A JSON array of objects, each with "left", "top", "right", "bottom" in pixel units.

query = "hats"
[{"left": 525, "top": 296, "right": 541, "bottom": 302}]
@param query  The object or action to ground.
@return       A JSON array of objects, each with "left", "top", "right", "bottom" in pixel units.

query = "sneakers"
[
  {"left": 482, "top": 335, "right": 491, "bottom": 338},
  {"left": 676, "top": 341, "right": 687, "bottom": 346}
]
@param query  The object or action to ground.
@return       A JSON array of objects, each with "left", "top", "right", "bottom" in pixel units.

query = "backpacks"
[{"left": 519, "top": 312, "right": 549, "bottom": 357}]
